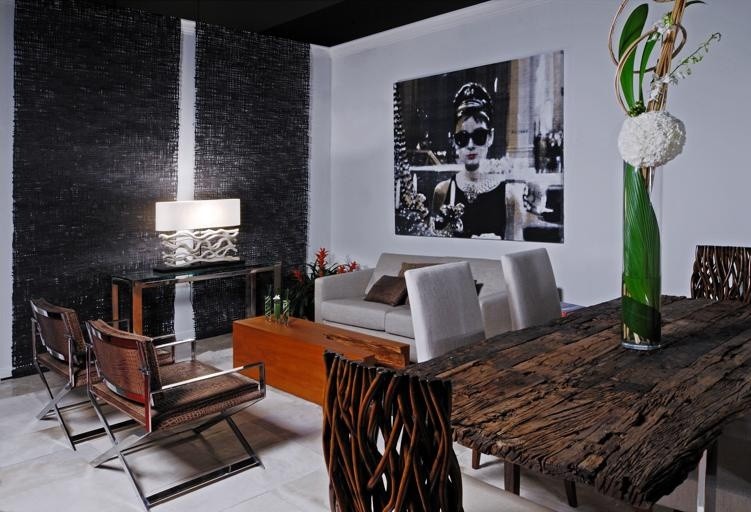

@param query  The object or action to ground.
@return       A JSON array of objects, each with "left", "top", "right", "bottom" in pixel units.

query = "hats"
[{"left": 451, "top": 81, "right": 495, "bottom": 116}]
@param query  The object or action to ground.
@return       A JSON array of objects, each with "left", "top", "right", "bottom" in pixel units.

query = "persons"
[{"left": 430, "top": 81, "right": 525, "bottom": 241}]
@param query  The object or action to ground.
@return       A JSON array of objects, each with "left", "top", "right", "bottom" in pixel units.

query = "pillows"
[
  {"left": 363, "top": 274, "right": 410, "bottom": 307},
  {"left": 397, "top": 263, "right": 439, "bottom": 279}
]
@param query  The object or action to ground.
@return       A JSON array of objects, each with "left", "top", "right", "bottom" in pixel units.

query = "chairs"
[
  {"left": 501, "top": 248, "right": 561, "bottom": 329},
  {"left": 689, "top": 245, "right": 751, "bottom": 300},
  {"left": 321, "top": 351, "right": 555, "bottom": 512},
  {"left": 402, "top": 263, "right": 487, "bottom": 469},
  {"left": 29, "top": 299, "right": 194, "bottom": 450},
  {"left": 80, "top": 318, "right": 266, "bottom": 512}
]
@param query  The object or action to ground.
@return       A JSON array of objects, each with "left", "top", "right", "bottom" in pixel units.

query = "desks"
[
  {"left": 110, "top": 261, "right": 282, "bottom": 335},
  {"left": 400, "top": 294, "right": 751, "bottom": 512}
]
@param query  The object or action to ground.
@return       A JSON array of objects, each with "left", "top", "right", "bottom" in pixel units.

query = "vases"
[{"left": 622, "top": 159, "right": 662, "bottom": 350}]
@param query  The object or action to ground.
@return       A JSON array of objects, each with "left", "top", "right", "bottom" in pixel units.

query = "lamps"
[{"left": 153, "top": 198, "right": 241, "bottom": 272}]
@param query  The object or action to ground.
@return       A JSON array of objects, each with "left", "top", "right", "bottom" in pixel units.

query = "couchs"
[{"left": 313, "top": 252, "right": 507, "bottom": 363}]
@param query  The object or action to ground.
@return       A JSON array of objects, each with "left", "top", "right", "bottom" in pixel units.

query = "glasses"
[{"left": 452, "top": 128, "right": 491, "bottom": 148}]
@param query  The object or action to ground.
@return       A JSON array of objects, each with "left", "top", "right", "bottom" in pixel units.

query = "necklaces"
[{"left": 465, "top": 163, "right": 480, "bottom": 172}]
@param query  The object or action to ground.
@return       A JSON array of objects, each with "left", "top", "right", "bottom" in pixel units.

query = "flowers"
[
  {"left": 606, "top": 1, "right": 723, "bottom": 344},
  {"left": 293, "top": 248, "right": 358, "bottom": 321}
]
